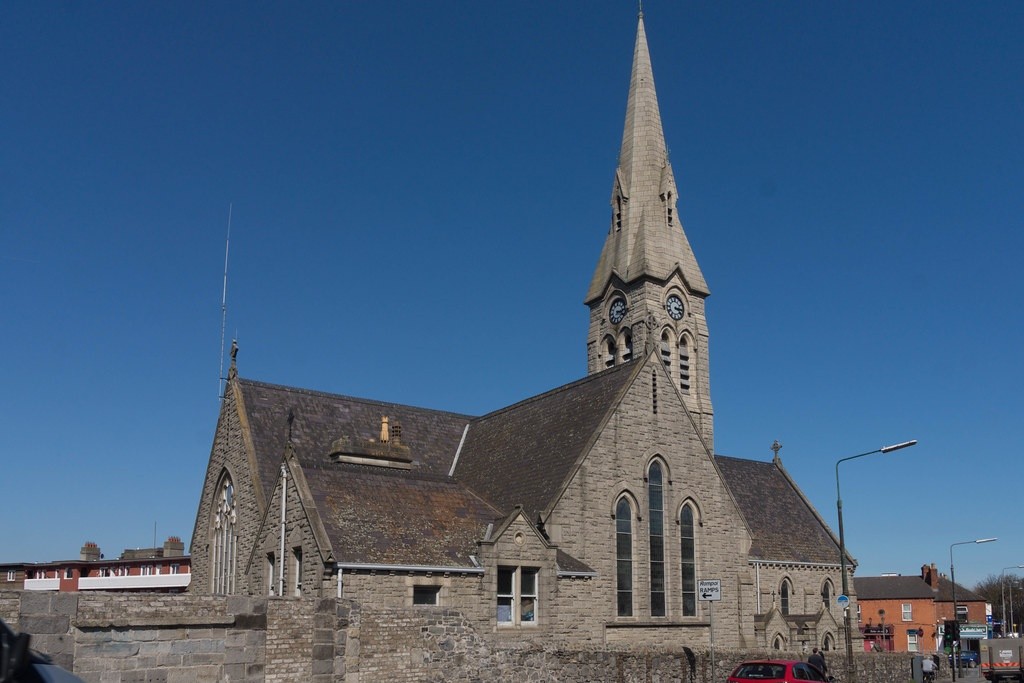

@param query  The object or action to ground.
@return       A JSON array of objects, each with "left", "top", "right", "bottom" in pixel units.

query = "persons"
[
  {"left": 808, "top": 648, "right": 827, "bottom": 679},
  {"left": 922, "top": 657, "right": 938, "bottom": 683}
]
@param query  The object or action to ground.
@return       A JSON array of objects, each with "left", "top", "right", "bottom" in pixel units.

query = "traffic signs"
[{"left": 697, "top": 579, "right": 722, "bottom": 601}]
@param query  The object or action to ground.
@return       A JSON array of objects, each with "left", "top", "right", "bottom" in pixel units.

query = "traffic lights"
[
  {"left": 954, "top": 620, "right": 960, "bottom": 641},
  {"left": 944, "top": 619, "right": 954, "bottom": 642}
]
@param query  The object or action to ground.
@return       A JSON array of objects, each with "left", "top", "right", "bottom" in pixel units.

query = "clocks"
[
  {"left": 666, "top": 296, "right": 684, "bottom": 320},
  {"left": 609, "top": 300, "right": 626, "bottom": 324}
]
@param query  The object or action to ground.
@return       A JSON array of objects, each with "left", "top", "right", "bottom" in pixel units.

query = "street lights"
[
  {"left": 949, "top": 537, "right": 999, "bottom": 682},
  {"left": 1001, "top": 564, "right": 1024, "bottom": 637},
  {"left": 835, "top": 439, "right": 917, "bottom": 683}
]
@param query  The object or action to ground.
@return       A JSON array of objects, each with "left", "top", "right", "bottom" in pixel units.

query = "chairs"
[{"left": 762, "top": 667, "right": 773, "bottom": 677}]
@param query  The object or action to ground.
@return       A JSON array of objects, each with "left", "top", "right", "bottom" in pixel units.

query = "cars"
[
  {"left": 946, "top": 650, "right": 981, "bottom": 668},
  {"left": 0, "top": 616, "right": 87, "bottom": 683},
  {"left": 725, "top": 658, "right": 834, "bottom": 683}
]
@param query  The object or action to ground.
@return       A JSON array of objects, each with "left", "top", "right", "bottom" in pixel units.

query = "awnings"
[{"left": 957, "top": 624, "right": 987, "bottom": 640}]
